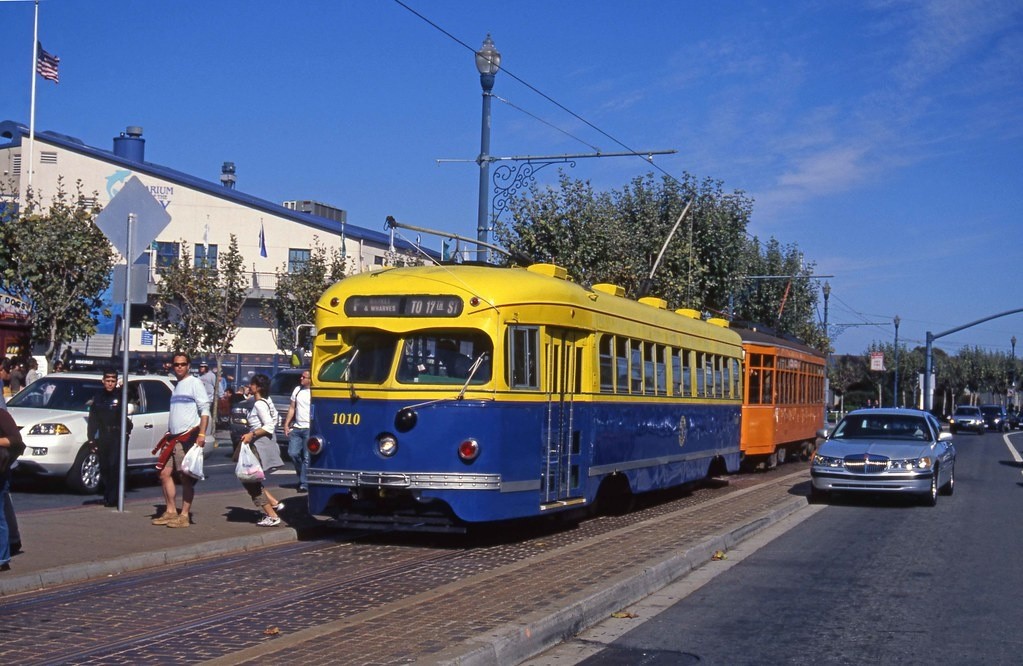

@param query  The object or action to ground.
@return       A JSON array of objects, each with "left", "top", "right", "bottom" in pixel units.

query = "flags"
[
  {"left": 203, "top": 224, "right": 210, "bottom": 248},
  {"left": 341, "top": 232, "right": 346, "bottom": 256},
  {"left": 37, "top": 42, "right": 59, "bottom": 83},
  {"left": 259, "top": 228, "right": 267, "bottom": 258}
]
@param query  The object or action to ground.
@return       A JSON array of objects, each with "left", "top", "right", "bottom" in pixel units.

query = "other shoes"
[{"left": 299, "top": 487, "right": 307, "bottom": 493}]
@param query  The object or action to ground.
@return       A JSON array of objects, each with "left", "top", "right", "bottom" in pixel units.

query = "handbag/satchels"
[
  {"left": 180, "top": 444, "right": 204, "bottom": 480},
  {"left": 233, "top": 438, "right": 266, "bottom": 482}
]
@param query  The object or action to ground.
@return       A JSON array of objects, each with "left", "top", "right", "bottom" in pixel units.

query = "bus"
[{"left": 290, "top": 217, "right": 828, "bottom": 536}]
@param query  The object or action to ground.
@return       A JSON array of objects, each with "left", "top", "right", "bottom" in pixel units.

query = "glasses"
[
  {"left": 173, "top": 362, "right": 187, "bottom": 366},
  {"left": 301, "top": 375, "right": 309, "bottom": 379}
]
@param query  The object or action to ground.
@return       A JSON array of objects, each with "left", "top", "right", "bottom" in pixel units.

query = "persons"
[
  {"left": 224, "top": 387, "right": 251, "bottom": 400},
  {"left": 865, "top": 399, "right": 879, "bottom": 408},
  {"left": 72, "top": 347, "right": 82, "bottom": 355},
  {"left": 239, "top": 374, "right": 284, "bottom": 526},
  {"left": 0, "top": 356, "right": 41, "bottom": 412},
  {"left": 0, "top": 408, "right": 21, "bottom": 570},
  {"left": 283, "top": 371, "right": 311, "bottom": 492},
  {"left": 198, "top": 361, "right": 227, "bottom": 412},
  {"left": 61, "top": 346, "right": 72, "bottom": 364},
  {"left": 439, "top": 340, "right": 477, "bottom": 378},
  {"left": 151, "top": 354, "right": 210, "bottom": 528},
  {"left": 87, "top": 370, "right": 140, "bottom": 507},
  {"left": 53, "top": 361, "right": 68, "bottom": 372}
]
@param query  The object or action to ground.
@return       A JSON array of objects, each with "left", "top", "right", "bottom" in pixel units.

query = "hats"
[{"left": 200, "top": 361, "right": 208, "bottom": 367}]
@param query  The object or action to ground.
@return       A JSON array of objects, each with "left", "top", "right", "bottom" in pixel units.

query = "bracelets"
[
  {"left": 198, "top": 434, "right": 205, "bottom": 436},
  {"left": 253, "top": 432, "right": 256, "bottom": 438}
]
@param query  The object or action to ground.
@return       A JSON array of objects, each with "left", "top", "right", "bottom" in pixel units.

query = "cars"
[
  {"left": 946, "top": 405, "right": 986, "bottom": 435},
  {"left": 978, "top": 404, "right": 1023, "bottom": 433},
  {"left": 810, "top": 408, "right": 957, "bottom": 507}
]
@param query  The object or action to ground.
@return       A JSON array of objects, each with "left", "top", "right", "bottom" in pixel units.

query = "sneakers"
[
  {"left": 271, "top": 502, "right": 284, "bottom": 512},
  {"left": 152, "top": 512, "right": 178, "bottom": 525},
  {"left": 166, "top": 513, "right": 190, "bottom": 528},
  {"left": 257, "top": 515, "right": 281, "bottom": 527}
]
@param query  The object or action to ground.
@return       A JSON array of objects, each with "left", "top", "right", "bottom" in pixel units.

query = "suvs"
[
  {"left": 229, "top": 366, "right": 310, "bottom": 451},
  {"left": 5, "top": 369, "right": 177, "bottom": 496}
]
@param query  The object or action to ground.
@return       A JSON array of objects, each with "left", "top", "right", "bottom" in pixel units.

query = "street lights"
[
  {"left": 474, "top": 33, "right": 502, "bottom": 262},
  {"left": 893, "top": 315, "right": 901, "bottom": 407},
  {"left": 1010, "top": 335, "right": 1018, "bottom": 360},
  {"left": 821, "top": 280, "right": 831, "bottom": 354}
]
